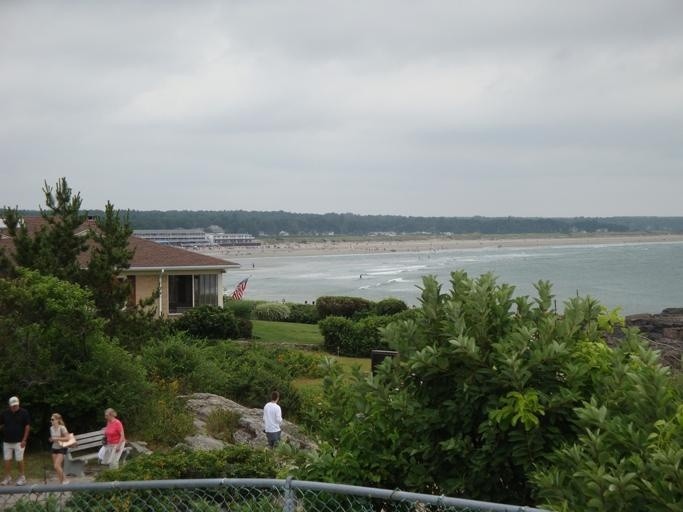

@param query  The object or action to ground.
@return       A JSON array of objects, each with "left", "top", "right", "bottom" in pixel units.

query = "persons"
[
  {"left": 0, "top": 396, "right": 29, "bottom": 486},
  {"left": 262, "top": 391, "right": 282, "bottom": 449},
  {"left": 99, "top": 407, "right": 126, "bottom": 470},
  {"left": 47, "top": 412, "right": 69, "bottom": 483}
]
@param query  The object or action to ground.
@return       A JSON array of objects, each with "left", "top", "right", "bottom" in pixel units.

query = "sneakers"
[
  {"left": 15, "top": 476, "right": 26, "bottom": 486},
  {"left": 62, "top": 479, "right": 70, "bottom": 486},
  {"left": 0, "top": 476, "right": 13, "bottom": 485}
]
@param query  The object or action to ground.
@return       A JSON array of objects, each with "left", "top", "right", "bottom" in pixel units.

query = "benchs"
[{"left": 61, "top": 430, "right": 132, "bottom": 477}]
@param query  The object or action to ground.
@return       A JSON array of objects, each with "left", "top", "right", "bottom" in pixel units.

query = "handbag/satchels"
[
  {"left": 101, "top": 436, "right": 108, "bottom": 446},
  {"left": 56, "top": 431, "right": 76, "bottom": 449}
]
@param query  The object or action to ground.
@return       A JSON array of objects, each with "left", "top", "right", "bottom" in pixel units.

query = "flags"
[{"left": 231, "top": 277, "right": 247, "bottom": 301}]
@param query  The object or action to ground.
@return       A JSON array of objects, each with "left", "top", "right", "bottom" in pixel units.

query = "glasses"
[{"left": 49, "top": 418, "right": 54, "bottom": 421}]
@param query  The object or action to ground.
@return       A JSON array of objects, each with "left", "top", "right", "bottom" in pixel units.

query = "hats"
[{"left": 7, "top": 396, "right": 19, "bottom": 406}]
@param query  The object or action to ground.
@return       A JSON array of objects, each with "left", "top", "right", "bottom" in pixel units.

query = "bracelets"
[{"left": 22, "top": 439, "right": 26, "bottom": 441}]
